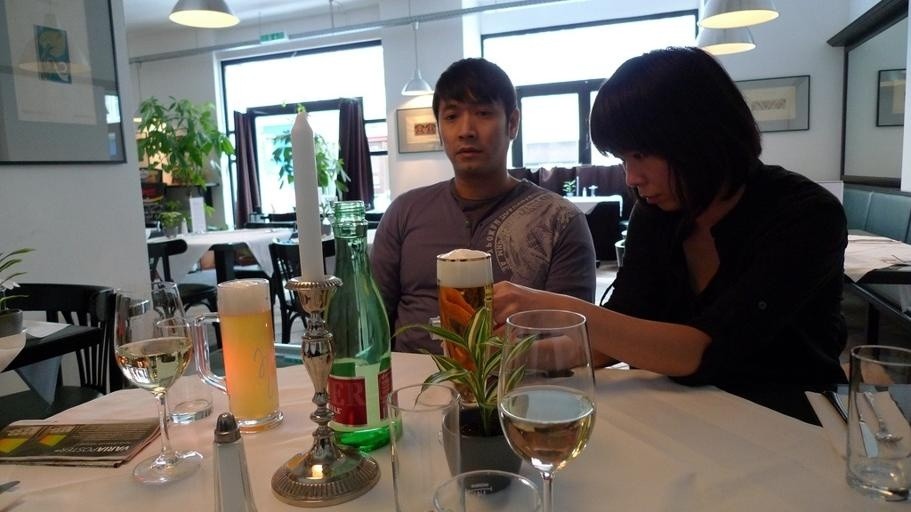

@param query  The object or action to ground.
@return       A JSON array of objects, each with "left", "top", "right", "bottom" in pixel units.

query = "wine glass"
[
  {"left": 114, "top": 280, "right": 203, "bottom": 483},
  {"left": 497, "top": 307, "right": 596, "bottom": 511}
]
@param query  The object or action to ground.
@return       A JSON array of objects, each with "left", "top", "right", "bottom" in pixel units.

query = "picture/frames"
[
  {"left": 875, "top": 67, "right": 905, "bottom": 127},
  {"left": 0, "top": 0, "right": 129, "bottom": 170},
  {"left": 395, "top": 106, "right": 445, "bottom": 154},
  {"left": 730, "top": 74, "right": 810, "bottom": 134}
]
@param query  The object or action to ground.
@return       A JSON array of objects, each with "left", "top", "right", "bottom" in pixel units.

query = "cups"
[
  {"left": 195, "top": 276, "right": 284, "bottom": 437},
  {"left": 844, "top": 341, "right": 911, "bottom": 503},
  {"left": 437, "top": 250, "right": 498, "bottom": 405},
  {"left": 431, "top": 468, "right": 544, "bottom": 510},
  {"left": 155, "top": 314, "right": 216, "bottom": 422},
  {"left": 388, "top": 384, "right": 468, "bottom": 512}
]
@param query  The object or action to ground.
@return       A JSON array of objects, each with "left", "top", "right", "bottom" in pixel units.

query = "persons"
[
  {"left": 440, "top": 47, "right": 849, "bottom": 427},
  {"left": 367, "top": 57, "right": 596, "bottom": 356}
]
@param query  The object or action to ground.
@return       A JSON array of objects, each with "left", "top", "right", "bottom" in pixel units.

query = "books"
[{"left": 0, "top": 417, "right": 170, "bottom": 469}]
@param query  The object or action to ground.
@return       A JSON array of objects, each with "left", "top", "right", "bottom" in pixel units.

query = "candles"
[{"left": 290, "top": 104, "right": 325, "bottom": 276}]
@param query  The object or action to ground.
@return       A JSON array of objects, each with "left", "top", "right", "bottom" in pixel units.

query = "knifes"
[{"left": 822, "top": 390, "right": 879, "bottom": 458}]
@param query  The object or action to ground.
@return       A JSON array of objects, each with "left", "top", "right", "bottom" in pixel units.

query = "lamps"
[
  {"left": 696, "top": 26, "right": 757, "bottom": 56},
  {"left": 400, "top": 20, "right": 432, "bottom": 94},
  {"left": 695, "top": 0, "right": 780, "bottom": 28},
  {"left": 166, "top": 0, "right": 240, "bottom": 30}
]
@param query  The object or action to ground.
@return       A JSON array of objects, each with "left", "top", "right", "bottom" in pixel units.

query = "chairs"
[{"left": 0, "top": 157, "right": 910, "bottom": 509}]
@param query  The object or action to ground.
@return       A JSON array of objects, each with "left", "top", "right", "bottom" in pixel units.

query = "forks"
[{"left": 861, "top": 388, "right": 903, "bottom": 442}]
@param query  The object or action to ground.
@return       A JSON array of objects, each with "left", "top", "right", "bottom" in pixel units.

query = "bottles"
[
  {"left": 324, "top": 198, "right": 403, "bottom": 451},
  {"left": 582, "top": 188, "right": 587, "bottom": 198},
  {"left": 181, "top": 218, "right": 188, "bottom": 237},
  {"left": 211, "top": 414, "right": 258, "bottom": 511}
]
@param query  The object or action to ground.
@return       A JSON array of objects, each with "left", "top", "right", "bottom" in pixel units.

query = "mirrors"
[{"left": 838, "top": 11, "right": 909, "bottom": 189}]
